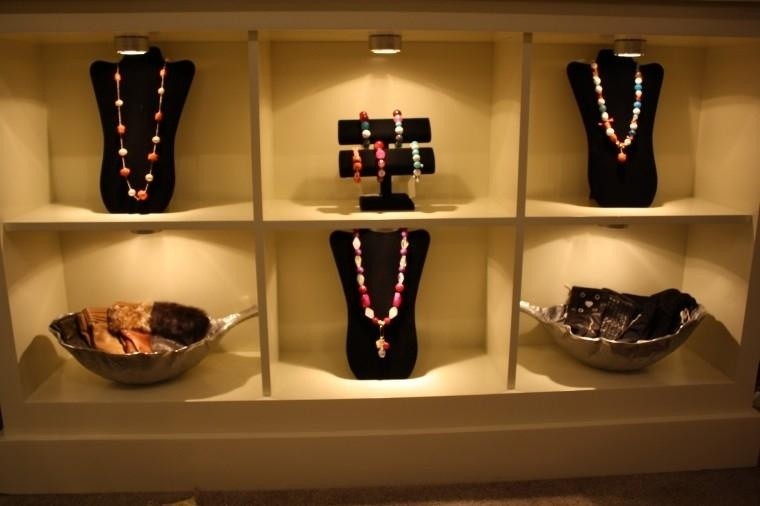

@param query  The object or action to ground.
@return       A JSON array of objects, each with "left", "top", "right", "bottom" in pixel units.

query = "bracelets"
[
  {"left": 375, "top": 141, "right": 386, "bottom": 183},
  {"left": 360, "top": 111, "right": 371, "bottom": 149},
  {"left": 352, "top": 148, "right": 363, "bottom": 184},
  {"left": 411, "top": 140, "right": 424, "bottom": 181},
  {"left": 393, "top": 109, "right": 404, "bottom": 148}
]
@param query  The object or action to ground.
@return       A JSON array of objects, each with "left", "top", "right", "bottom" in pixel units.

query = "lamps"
[
  {"left": 611, "top": 37, "right": 648, "bottom": 58},
  {"left": 369, "top": 33, "right": 401, "bottom": 53},
  {"left": 114, "top": 35, "right": 148, "bottom": 54}
]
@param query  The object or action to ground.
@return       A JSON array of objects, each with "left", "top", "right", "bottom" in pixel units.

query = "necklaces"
[
  {"left": 592, "top": 58, "right": 643, "bottom": 162},
  {"left": 115, "top": 64, "right": 167, "bottom": 201},
  {"left": 352, "top": 228, "right": 409, "bottom": 360}
]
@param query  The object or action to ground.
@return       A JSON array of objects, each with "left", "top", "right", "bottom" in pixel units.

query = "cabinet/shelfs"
[{"left": 0, "top": 8, "right": 759, "bottom": 493}]
